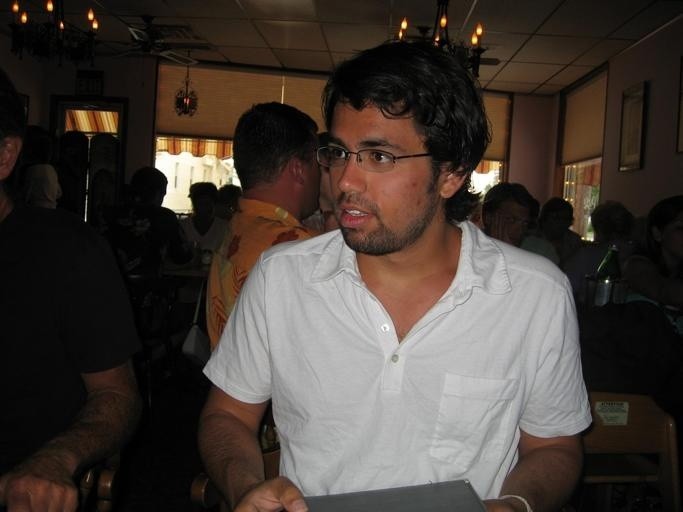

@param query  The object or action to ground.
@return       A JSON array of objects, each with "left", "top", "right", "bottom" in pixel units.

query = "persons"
[
  {"left": 196, "top": 37, "right": 596, "bottom": 511},
  {"left": 206, "top": 101, "right": 321, "bottom": 349},
  {"left": 500, "top": 495, "right": 533, "bottom": 512},
  {"left": 0, "top": 69, "right": 144, "bottom": 511}
]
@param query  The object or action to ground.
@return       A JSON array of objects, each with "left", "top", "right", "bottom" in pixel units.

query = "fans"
[{"left": 94, "top": 17, "right": 210, "bottom": 67}]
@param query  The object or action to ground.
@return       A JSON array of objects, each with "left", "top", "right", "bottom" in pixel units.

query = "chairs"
[
  {"left": 580, "top": 390, "right": 681, "bottom": 512},
  {"left": 183, "top": 421, "right": 281, "bottom": 511},
  {"left": 76, "top": 421, "right": 132, "bottom": 509}
]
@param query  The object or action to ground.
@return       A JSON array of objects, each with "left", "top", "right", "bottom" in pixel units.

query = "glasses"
[{"left": 314, "top": 144, "right": 439, "bottom": 173}]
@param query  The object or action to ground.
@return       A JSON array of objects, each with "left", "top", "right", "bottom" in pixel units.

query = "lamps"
[
  {"left": 0, "top": 0, "right": 102, "bottom": 72},
  {"left": 174, "top": 50, "right": 198, "bottom": 117},
  {"left": 394, "top": 1, "right": 501, "bottom": 77}
]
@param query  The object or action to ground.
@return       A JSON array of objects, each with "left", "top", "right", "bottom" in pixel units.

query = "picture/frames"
[{"left": 616, "top": 79, "right": 649, "bottom": 174}]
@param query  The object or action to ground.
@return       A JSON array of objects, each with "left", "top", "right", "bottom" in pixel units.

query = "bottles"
[{"left": 591, "top": 242, "right": 622, "bottom": 308}]
